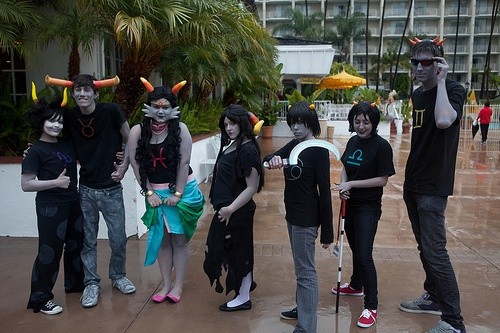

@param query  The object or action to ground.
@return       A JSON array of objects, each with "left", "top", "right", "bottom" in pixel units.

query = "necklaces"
[{"left": 149, "top": 119, "right": 170, "bottom": 140}]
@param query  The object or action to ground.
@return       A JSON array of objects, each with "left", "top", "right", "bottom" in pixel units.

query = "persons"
[
  {"left": 330, "top": 99, "right": 396, "bottom": 329},
  {"left": 202, "top": 101, "right": 266, "bottom": 312},
  {"left": 477, "top": 101, "right": 493, "bottom": 142},
  {"left": 398, "top": 32, "right": 470, "bottom": 332},
  {"left": 20, "top": 81, "right": 88, "bottom": 316},
  {"left": 127, "top": 76, "right": 205, "bottom": 305},
  {"left": 21, "top": 73, "right": 138, "bottom": 308},
  {"left": 261, "top": 99, "right": 335, "bottom": 332}
]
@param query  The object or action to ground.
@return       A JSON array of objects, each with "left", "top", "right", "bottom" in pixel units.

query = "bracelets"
[
  {"left": 147, "top": 189, "right": 154, "bottom": 196},
  {"left": 174, "top": 190, "right": 182, "bottom": 197}
]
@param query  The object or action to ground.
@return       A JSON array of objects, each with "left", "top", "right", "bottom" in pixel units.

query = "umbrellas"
[
  {"left": 322, "top": 70, "right": 367, "bottom": 104},
  {"left": 471, "top": 121, "right": 479, "bottom": 139}
]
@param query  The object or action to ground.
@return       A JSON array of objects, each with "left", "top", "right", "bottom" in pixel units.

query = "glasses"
[{"left": 411, "top": 59, "right": 438, "bottom": 66}]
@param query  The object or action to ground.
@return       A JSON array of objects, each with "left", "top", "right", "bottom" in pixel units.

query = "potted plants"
[
  {"left": 394, "top": 100, "right": 403, "bottom": 133},
  {"left": 460, "top": 114, "right": 474, "bottom": 130},
  {"left": 259, "top": 101, "right": 283, "bottom": 139}
]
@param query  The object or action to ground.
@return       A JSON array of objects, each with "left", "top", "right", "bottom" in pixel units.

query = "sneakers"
[
  {"left": 112, "top": 278, "right": 136, "bottom": 294},
  {"left": 34, "top": 300, "right": 63, "bottom": 315},
  {"left": 66, "top": 285, "right": 85, "bottom": 293},
  {"left": 281, "top": 307, "right": 298, "bottom": 320},
  {"left": 332, "top": 283, "right": 364, "bottom": 296},
  {"left": 80, "top": 285, "right": 100, "bottom": 307},
  {"left": 399, "top": 293, "right": 442, "bottom": 315},
  {"left": 357, "top": 309, "right": 378, "bottom": 327},
  {"left": 427, "top": 321, "right": 466, "bottom": 333}
]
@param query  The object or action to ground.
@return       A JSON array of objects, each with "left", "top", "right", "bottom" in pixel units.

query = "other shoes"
[
  {"left": 167, "top": 290, "right": 183, "bottom": 302},
  {"left": 234, "top": 281, "right": 257, "bottom": 299},
  {"left": 218, "top": 299, "right": 252, "bottom": 311},
  {"left": 152, "top": 289, "right": 171, "bottom": 302}
]
